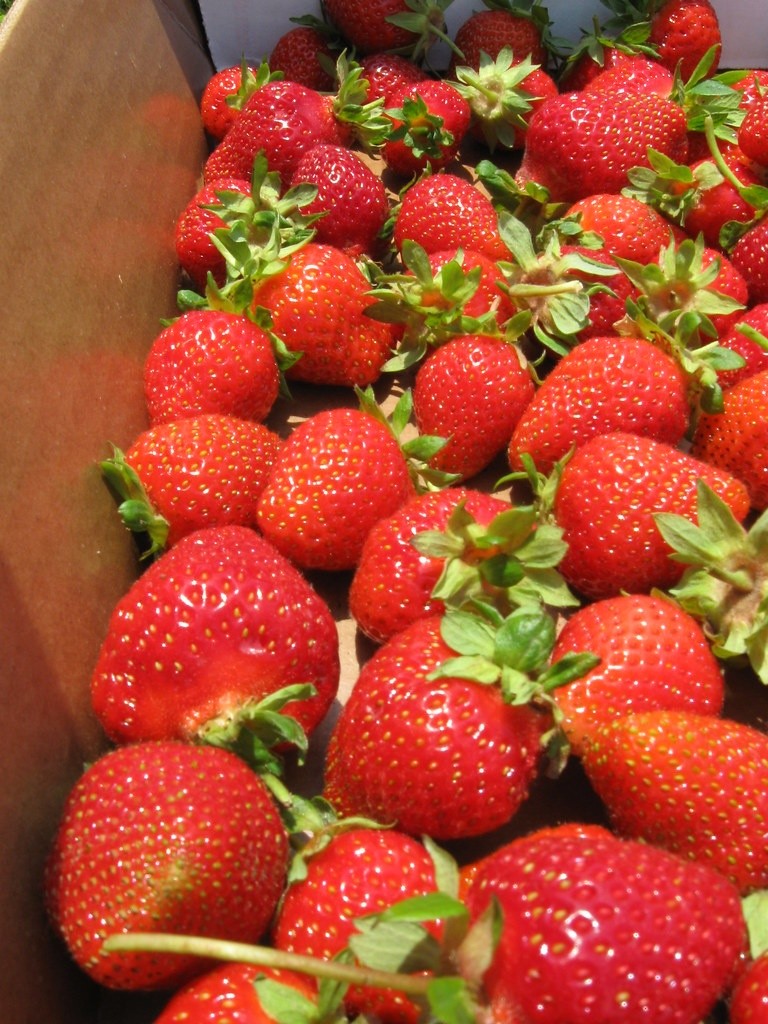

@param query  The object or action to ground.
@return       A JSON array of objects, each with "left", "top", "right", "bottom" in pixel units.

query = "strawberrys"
[{"left": 43, "top": 0, "right": 768, "bottom": 1024}]
